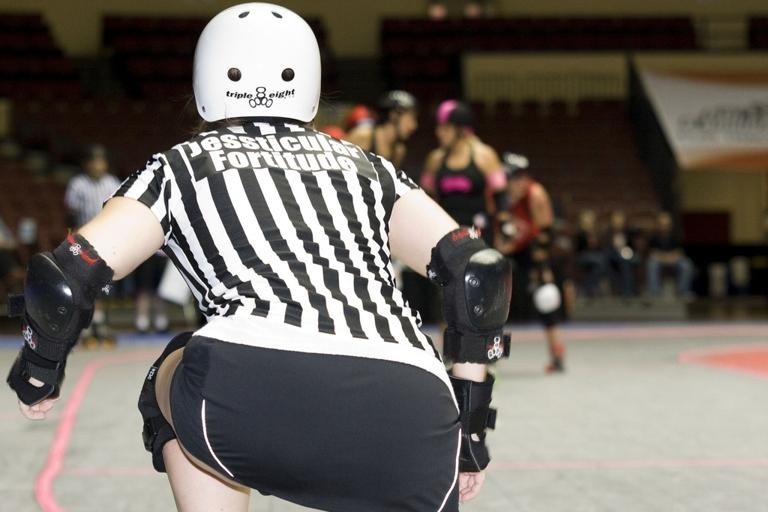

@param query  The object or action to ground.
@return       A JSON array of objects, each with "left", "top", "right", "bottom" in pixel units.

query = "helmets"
[
  {"left": 193, "top": 2, "right": 322, "bottom": 124},
  {"left": 373, "top": 90, "right": 416, "bottom": 109},
  {"left": 434, "top": 100, "right": 473, "bottom": 125}
]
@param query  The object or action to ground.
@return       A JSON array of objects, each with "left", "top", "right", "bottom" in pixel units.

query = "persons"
[
  {"left": 322, "top": 90, "right": 418, "bottom": 170},
  {"left": 1, "top": 1, "right": 519, "bottom": 512},
  {"left": 482, "top": 152, "right": 571, "bottom": 373},
  {"left": 571, "top": 208, "right": 692, "bottom": 296},
  {"left": 134, "top": 248, "right": 173, "bottom": 335},
  {"left": 64, "top": 144, "right": 121, "bottom": 349},
  {"left": 418, "top": 99, "right": 516, "bottom": 250}
]
